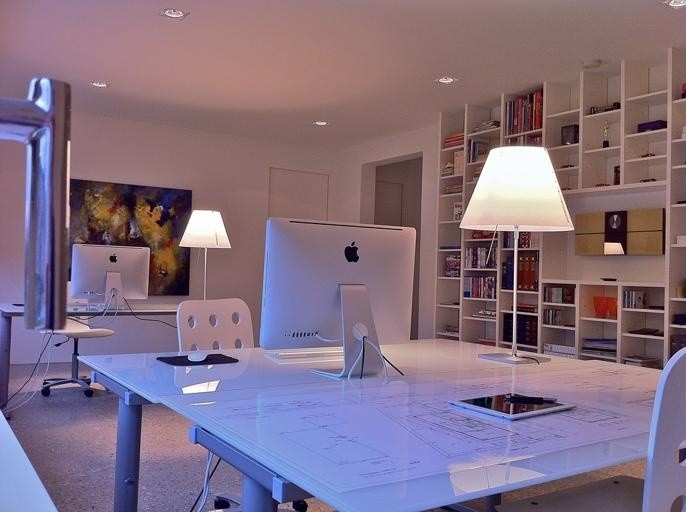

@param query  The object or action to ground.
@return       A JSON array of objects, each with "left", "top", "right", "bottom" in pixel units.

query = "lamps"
[
  {"left": 178, "top": 210, "right": 232, "bottom": 300},
  {"left": 459, "top": 146, "right": 575, "bottom": 367}
]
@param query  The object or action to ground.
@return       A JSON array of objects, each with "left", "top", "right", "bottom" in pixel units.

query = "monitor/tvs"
[
  {"left": 72, "top": 243, "right": 151, "bottom": 311},
  {"left": 258, "top": 215, "right": 416, "bottom": 380}
]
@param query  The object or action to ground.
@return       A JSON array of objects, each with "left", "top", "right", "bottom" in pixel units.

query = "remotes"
[
  {"left": 600, "top": 276, "right": 616, "bottom": 282},
  {"left": 12, "top": 303, "right": 24, "bottom": 307}
]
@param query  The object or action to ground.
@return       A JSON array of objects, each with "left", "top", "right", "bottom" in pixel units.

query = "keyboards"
[{"left": 270, "top": 350, "right": 342, "bottom": 359}]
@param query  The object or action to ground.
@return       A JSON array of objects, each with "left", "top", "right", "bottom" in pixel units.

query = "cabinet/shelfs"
[{"left": 433, "top": 46, "right": 686, "bottom": 371}]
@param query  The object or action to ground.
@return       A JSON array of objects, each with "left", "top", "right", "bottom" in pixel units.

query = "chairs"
[
  {"left": 176, "top": 297, "right": 308, "bottom": 512},
  {"left": 41, "top": 279, "right": 115, "bottom": 397}
]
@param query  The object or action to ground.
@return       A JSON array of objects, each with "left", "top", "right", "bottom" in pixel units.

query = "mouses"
[{"left": 187, "top": 350, "right": 209, "bottom": 363}]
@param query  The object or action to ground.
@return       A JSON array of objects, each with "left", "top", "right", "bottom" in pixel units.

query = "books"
[
  {"left": 440, "top": 150, "right": 465, "bottom": 193},
  {"left": 444, "top": 254, "right": 461, "bottom": 277},
  {"left": 619, "top": 353, "right": 660, "bottom": 369},
  {"left": 502, "top": 88, "right": 543, "bottom": 146},
  {"left": 464, "top": 275, "right": 496, "bottom": 299},
  {"left": 511, "top": 303, "right": 538, "bottom": 313},
  {"left": 472, "top": 119, "right": 500, "bottom": 133},
  {"left": 582, "top": 336, "right": 617, "bottom": 358},
  {"left": 502, "top": 257, "right": 537, "bottom": 291},
  {"left": 444, "top": 133, "right": 464, "bottom": 149},
  {"left": 464, "top": 246, "right": 496, "bottom": 268},
  {"left": 543, "top": 286, "right": 563, "bottom": 303},
  {"left": 624, "top": 290, "right": 644, "bottom": 309},
  {"left": 469, "top": 139, "right": 489, "bottom": 163},
  {"left": 503, "top": 314, "right": 537, "bottom": 348},
  {"left": 544, "top": 308, "right": 562, "bottom": 325}
]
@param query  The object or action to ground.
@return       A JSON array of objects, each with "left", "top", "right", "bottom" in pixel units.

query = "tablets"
[{"left": 446, "top": 390, "right": 576, "bottom": 422}]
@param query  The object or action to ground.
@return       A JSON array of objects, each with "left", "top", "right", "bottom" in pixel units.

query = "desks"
[{"left": 0, "top": 303, "right": 181, "bottom": 409}]
